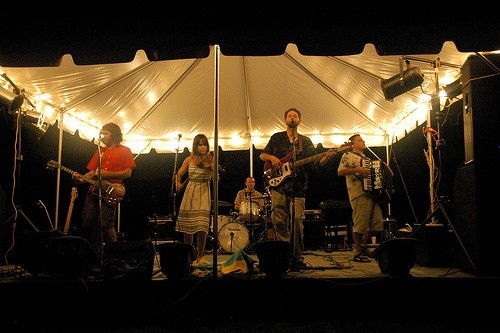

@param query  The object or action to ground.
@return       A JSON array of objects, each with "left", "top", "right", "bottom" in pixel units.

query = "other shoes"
[
  {"left": 354, "top": 253, "right": 371, "bottom": 262},
  {"left": 295, "top": 261, "right": 306, "bottom": 266},
  {"left": 198, "top": 258, "right": 210, "bottom": 264}
]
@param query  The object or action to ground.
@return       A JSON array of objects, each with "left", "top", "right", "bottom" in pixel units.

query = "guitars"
[
  {"left": 263, "top": 141, "right": 356, "bottom": 188},
  {"left": 45, "top": 157, "right": 125, "bottom": 206},
  {"left": 62, "top": 186, "right": 79, "bottom": 234}
]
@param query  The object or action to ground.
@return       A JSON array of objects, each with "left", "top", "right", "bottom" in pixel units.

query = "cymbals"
[
  {"left": 251, "top": 195, "right": 273, "bottom": 199},
  {"left": 259, "top": 203, "right": 272, "bottom": 207},
  {"left": 211, "top": 200, "right": 232, "bottom": 207}
]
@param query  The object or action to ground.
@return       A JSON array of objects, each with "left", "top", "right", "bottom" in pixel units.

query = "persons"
[
  {"left": 259, "top": 107, "right": 336, "bottom": 267},
  {"left": 175, "top": 134, "right": 220, "bottom": 259},
  {"left": 337, "top": 134, "right": 384, "bottom": 262},
  {"left": 234, "top": 177, "right": 264, "bottom": 211},
  {"left": 71, "top": 122, "right": 136, "bottom": 242}
]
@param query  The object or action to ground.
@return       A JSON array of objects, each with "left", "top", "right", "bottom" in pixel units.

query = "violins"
[{"left": 192, "top": 152, "right": 226, "bottom": 173}]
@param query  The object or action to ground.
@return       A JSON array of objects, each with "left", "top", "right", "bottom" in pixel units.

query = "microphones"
[
  {"left": 291, "top": 117, "right": 295, "bottom": 125},
  {"left": 230, "top": 232, "right": 234, "bottom": 236},
  {"left": 178, "top": 134, "right": 182, "bottom": 140},
  {"left": 245, "top": 192, "right": 248, "bottom": 200},
  {"left": 96, "top": 134, "right": 104, "bottom": 143}
]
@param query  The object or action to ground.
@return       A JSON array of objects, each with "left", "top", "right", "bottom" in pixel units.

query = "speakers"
[
  {"left": 370, "top": 238, "right": 422, "bottom": 277},
  {"left": 239, "top": 240, "right": 292, "bottom": 276},
  {"left": 17, "top": 219, "right": 192, "bottom": 276},
  {"left": 455, "top": 53, "right": 500, "bottom": 278}
]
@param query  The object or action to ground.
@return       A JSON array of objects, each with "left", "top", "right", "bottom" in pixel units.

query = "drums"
[
  {"left": 210, "top": 214, "right": 234, "bottom": 233},
  {"left": 238, "top": 200, "right": 261, "bottom": 222},
  {"left": 217, "top": 221, "right": 251, "bottom": 253}
]
